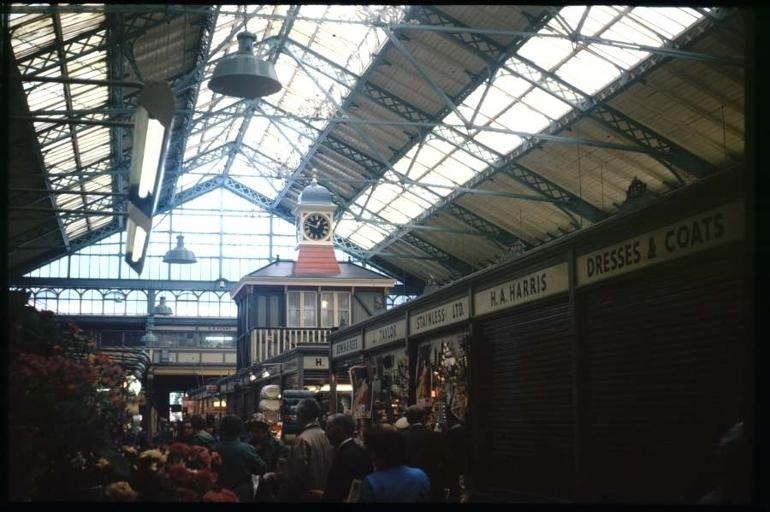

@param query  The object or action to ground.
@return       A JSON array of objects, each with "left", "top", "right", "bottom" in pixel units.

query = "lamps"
[
  {"left": 248, "top": 362, "right": 288, "bottom": 381},
  {"left": 208, "top": 5, "right": 282, "bottom": 97},
  {"left": 150, "top": 297, "right": 172, "bottom": 316},
  {"left": 163, "top": 123, "right": 197, "bottom": 264}
]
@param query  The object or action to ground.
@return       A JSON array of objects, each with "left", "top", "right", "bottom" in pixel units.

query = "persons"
[{"left": 157, "top": 392, "right": 467, "bottom": 503}]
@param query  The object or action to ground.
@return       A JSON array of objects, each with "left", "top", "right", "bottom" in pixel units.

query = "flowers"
[{"left": 8, "top": 309, "right": 241, "bottom": 503}]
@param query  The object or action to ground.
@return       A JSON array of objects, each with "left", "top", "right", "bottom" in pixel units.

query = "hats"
[{"left": 243, "top": 412, "right": 271, "bottom": 432}]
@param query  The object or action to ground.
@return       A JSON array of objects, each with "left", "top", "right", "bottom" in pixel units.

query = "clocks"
[{"left": 295, "top": 212, "right": 333, "bottom": 244}]
